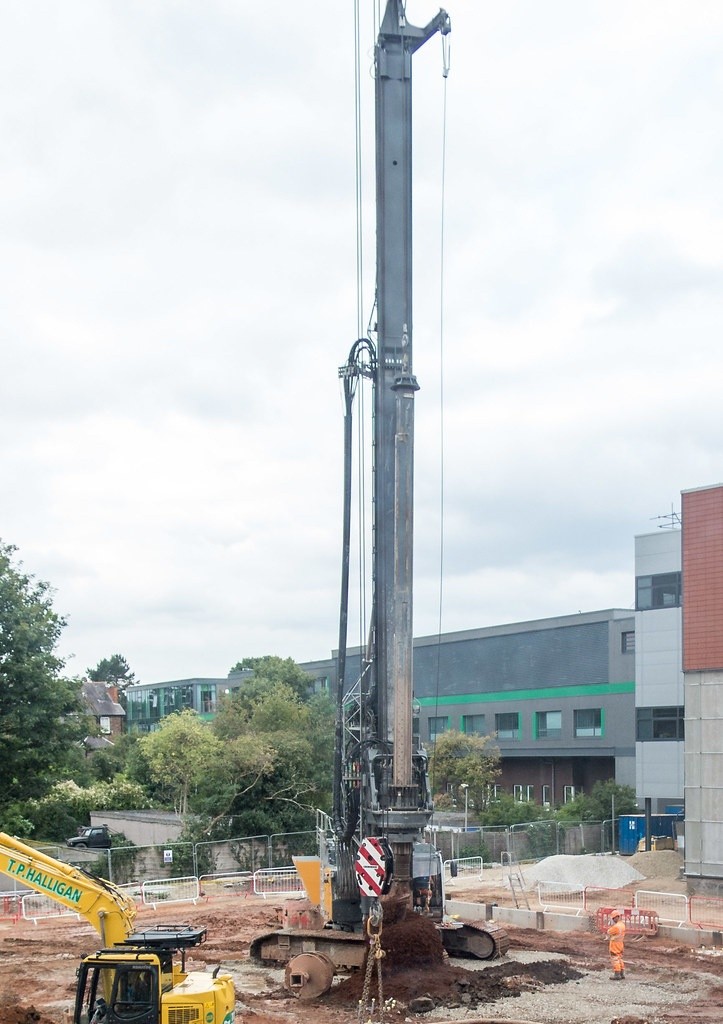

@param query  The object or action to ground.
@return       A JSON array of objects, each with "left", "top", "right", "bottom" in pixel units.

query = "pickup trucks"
[{"left": 66, "top": 826, "right": 127, "bottom": 850}]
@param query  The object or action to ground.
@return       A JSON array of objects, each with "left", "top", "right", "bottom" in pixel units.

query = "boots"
[{"left": 609, "top": 970, "right": 625, "bottom": 980}]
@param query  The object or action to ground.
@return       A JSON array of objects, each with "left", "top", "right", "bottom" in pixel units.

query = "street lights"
[{"left": 460, "top": 783, "right": 469, "bottom": 832}]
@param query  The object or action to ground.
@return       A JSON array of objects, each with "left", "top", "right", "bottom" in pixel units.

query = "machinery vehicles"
[
  {"left": 246, "top": 1, "right": 512, "bottom": 977},
  {"left": 0, "top": 830, "right": 238, "bottom": 1024}
]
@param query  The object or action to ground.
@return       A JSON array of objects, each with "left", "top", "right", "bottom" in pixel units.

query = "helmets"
[{"left": 611, "top": 910, "right": 620, "bottom": 919}]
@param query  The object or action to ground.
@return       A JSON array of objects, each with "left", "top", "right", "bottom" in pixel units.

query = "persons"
[{"left": 607, "top": 910, "right": 626, "bottom": 980}]
